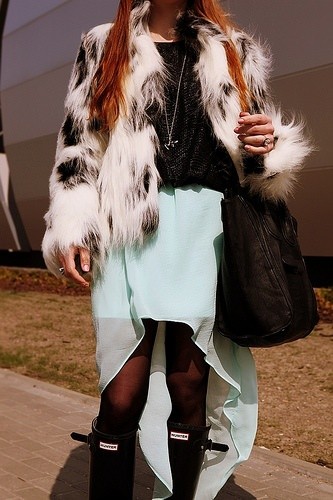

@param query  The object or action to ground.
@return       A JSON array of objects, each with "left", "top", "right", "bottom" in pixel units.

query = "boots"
[
  {"left": 71, "top": 418, "right": 141, "bottom": 500},
  {"left": 164, "top": 420, "right": 229, "bottom": 500}
]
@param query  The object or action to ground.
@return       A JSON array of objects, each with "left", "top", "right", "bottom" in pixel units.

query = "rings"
[
  {"left": 58, "top": 268, "right": 65, "bottom": 275},
  {"left": 263, "top": 135, "right": 271, "bottom": 146}
]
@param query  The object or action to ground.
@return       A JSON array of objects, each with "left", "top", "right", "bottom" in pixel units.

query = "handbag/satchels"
[{"left": 216, "top": 186, "right": 320, "bottom": 347}]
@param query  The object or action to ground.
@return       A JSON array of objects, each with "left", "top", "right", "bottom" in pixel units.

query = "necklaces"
[{"left": 154, "top": 51, "right": 187, "bottom": 150}]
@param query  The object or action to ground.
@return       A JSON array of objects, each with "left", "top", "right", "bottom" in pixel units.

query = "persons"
[{"left": 42, "top": 0, "right": 310, "bottom": 500}]
